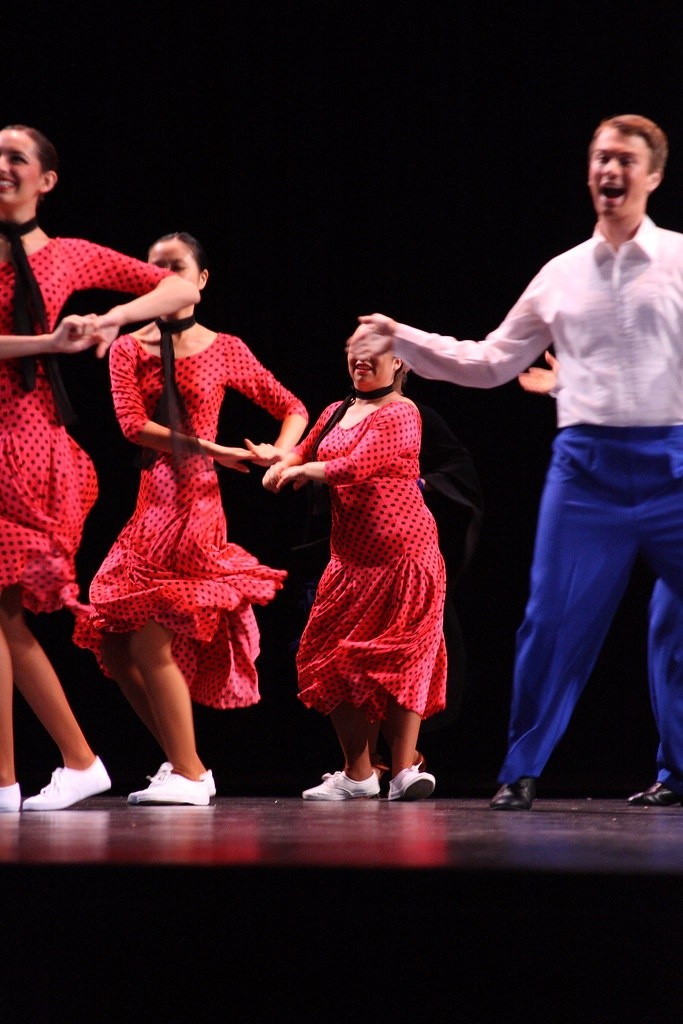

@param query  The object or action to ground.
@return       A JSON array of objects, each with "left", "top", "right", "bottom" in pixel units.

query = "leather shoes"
[
  {"left": 627, "top": 778, "right": 683, "bottom": 807},
  {"left": 489, "top": 775, "right": 534, "bottom": 811}
]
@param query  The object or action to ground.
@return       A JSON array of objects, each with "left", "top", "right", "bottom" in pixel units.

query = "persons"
[
  {"left": 518, "top": 350, "right": 683, "bottom": 808},
  {"left": 71, "top": 233, "right": 309, "bottom": 808},
  {"left": 0, "top": 122, "right": 200, "bottom": 813},
  {"left": 262, "top": 321, "right": 483, "bottom": 802},
  {"left": 345, "top": 114, "right": 683, "bottom": 814}
]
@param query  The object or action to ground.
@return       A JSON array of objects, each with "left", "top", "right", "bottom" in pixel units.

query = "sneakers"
[
  {"left": 387, "top": 760, "right": 436, "bottom": 800},
  {"left": 24, "top": 754, "right": 112, "bottom": 811},
  {"left": 0, "top": 782, "right": 22, "bottom": 814},
  {"left": 301, "top": 767, "right": 382, "bottom": 802},
  {"left": 127, "top": 764, "right": 218, "bottom": 807}
]
